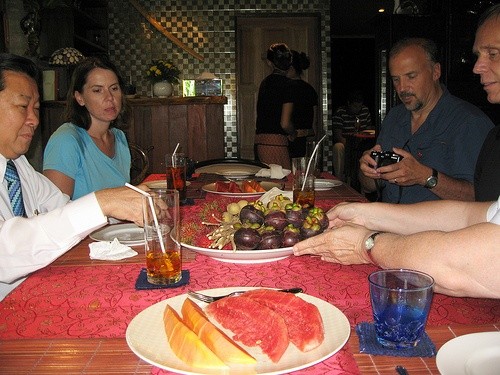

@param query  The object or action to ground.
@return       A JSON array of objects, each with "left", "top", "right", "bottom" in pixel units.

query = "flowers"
[
  {"left": 143, "top": 60, "right": 180, "bottom": 86},
  {"left": 47, "top": 47, "right": 86, "bottom": 98}
]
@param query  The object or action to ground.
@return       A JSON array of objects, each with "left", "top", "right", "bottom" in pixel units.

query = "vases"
[{"left": 154, "top": 79, "right": 172, "bottom": 98}]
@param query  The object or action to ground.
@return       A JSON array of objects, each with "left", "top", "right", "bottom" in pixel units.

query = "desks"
[
  {"left": 0, "top": 173, "right": 500, "bottom": 375},
  {"left": 343, "top": 131, "right": 378, "bottom": 193},
  {"left": 40, "top": 94, "right": 228, "bottom": 174}
]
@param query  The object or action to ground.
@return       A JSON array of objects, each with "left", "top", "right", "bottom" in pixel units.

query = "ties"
[{"left": 4, "top": 160, "right": 29, "bottom": 219}]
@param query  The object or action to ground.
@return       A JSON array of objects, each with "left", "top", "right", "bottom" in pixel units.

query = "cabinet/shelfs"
[{"left": 34, "top": 0, "right": 109, "bottom": 99}]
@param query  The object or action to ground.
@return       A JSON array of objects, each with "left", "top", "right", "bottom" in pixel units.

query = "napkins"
[
  {"left": 256, "top": 186, "right": 293, "bottom": 205},
  {"left": 87, "top": 238, "right": 139, "bottom": 261},
  {"left": 254, "top": 164, "right": 291, "bottom": 179}
]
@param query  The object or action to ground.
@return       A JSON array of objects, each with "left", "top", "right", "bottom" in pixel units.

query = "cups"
[
  {"left": 355, "top": 118, "right": 360, "bottom": 132},
  {"left": 166, "top": 153, "right": 187, "bottom": 199},
  {"left": 291, "top": 158, "right": 315, "bottom": 207},
  {"left": 369, "top": 268, "right": 436, "bottom": 350},
  {"left": 141, "top": 189, "right": 182, "bottom": 286}
]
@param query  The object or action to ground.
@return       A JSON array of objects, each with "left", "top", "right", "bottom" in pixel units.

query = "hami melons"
[
  {"left": 163, "top": 305, "right": 227, "bottom": 367},
  {"left": 242, "top": 178, "right": 266, "bottom": 192},
  {"left": 183, "top": 297, "right": 257, "bottom": 363}
]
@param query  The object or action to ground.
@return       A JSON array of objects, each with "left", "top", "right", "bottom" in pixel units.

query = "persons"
[
  {"left": 0, "top": 54, "right": 173, "bottom": 299},
  {"left": 291, "top": 3, "right": 500, "bottom": 301},
  {"left": 44, "top": 57, "right": 131, "bottom": 205},
  {"left": 255, "top": 43, "right": 371, "bottom": 181}
]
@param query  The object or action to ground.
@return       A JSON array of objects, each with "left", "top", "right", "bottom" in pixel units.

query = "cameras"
[{"left": 368, "top": 150, "right": 401, "bottom": 170}]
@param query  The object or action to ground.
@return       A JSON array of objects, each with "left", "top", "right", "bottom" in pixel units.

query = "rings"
[{"left": 395, "top": 178, "right": 397, "bottom": 183}]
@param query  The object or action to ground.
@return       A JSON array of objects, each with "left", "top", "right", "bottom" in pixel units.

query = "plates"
[
  {"left": 89, "top": 223, "right": 170, "bottom": 246},
  {"left": 218, "top": 173, "right": 254, "bottom": 180},
  {"left": 170, "top": 226, "right": 295, "bottom": 264},
  {"left": 143, "top": 179, "right": 191, "bottom": 189},
  {"left": 203, "top": 181, "right": 285, "bottom": 197},
  {"left": 435, "top": 331, "right": 500, "bottom": 375},
  {"left": 363, "top": 130, "right": 377, "bottom": 134},
  {"left": 126, "top": 286, "right": 351, "bottom": 375},
  {"left": 256, "top": 169, "right": 292, "bottom": 177},
  {"left": 308, "top": 179, "right": 342, "bottom": 191}
]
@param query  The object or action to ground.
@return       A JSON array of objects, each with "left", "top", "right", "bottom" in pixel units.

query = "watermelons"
[
  {"left": 204, "top": 296, "right": 290, "bottom": 364},
  {"left": 238, "top": 289, "right": 324, "bottom": 351},
  {"left": 215, "top": 179, "right": 242, "bottom": 193}
]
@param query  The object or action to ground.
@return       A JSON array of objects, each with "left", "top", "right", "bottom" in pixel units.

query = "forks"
[{"left": 188, "top": 288, "right": 303, "bottom": 304}]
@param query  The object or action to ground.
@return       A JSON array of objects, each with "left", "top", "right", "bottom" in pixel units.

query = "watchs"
[
  {"left": 365, "top": 231, "right": 384, "bottom": 266},
  {"left": 424, "top": 168, "right": 438, "bottom": 188}
]
